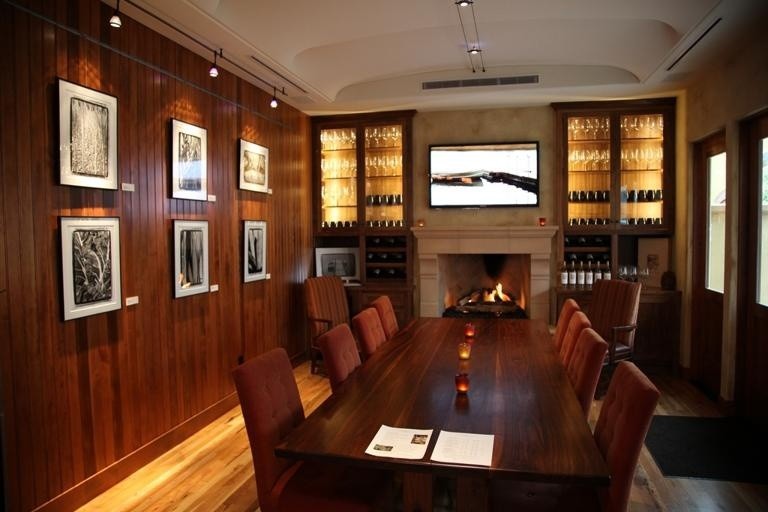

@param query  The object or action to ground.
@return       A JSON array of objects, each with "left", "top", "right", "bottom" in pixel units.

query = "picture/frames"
[
  {"left": 239, "top": 218, "right": 269, "bottom": 285},
  {"left": 637, "top": 237, "right": 670, "bottom": 288},
  {"left": 169, "top": 214, "right": 211, "bottom": 301},
  {"left": 56, "top": 212, "right": 125, "bottom": 322},
  {"left": 166, "top": 115, "right": 209, "bottom": 205},
  {"left": 315, "top": 247, "right": 360, "bottom": 282},
  {"left": 236, "top": 137, "right": 271, "bottom": 196},
  {"left": 56, "top": 76, "right": 122, "bottom": 192}
]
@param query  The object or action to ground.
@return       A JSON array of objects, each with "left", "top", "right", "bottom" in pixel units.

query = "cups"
[
  {"left": 568, "top": 188, "right": 663, "bottom": 202},
  {"left": 570, "top": 217, "right": 661, "bottom": 225},
  {"left": 416, "top": 217, "right": 424, "bottom": 228},
  {"left": 367, "top": 193, "right": 402, "bottom": 205},
  {"left": 539, "top": 216, "right": 546, "bottom": 227},
  {"left": 322, "top": 218, "right": 406, "bottom": 229},
  {"left": 454, "top": 373, "right": 469, "bottom": 393},
  {"left": 464, "top": 320, "right": 474, "bottom": 337},
  {"left": 457, "top": 342, "right": 471, "bottom": 360},
  {"left": 454, "top": 392, "right": 469, "bottom": 414}
]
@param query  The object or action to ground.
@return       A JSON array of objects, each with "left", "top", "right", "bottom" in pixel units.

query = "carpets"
[{"left": 646, "top": 405, "right": 767, "bottom": 488}]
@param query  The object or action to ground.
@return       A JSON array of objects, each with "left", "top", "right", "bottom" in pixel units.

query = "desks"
[
  {"left": 554, "top": 284, "right": 680, "bottom": 371},
  {"left": 273, "top": 313, "right": 614, "bottom": 512}
]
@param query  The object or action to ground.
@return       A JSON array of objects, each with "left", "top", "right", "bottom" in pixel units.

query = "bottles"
[
  {"left": 565, "top": 236, "right": 605, "bottom": 248},
  {"left": 560, "top": 261, "right": 611, "bottom": 288},
  {"left": 366, "top": 237, "right": 407, "bottom": 278},
  {"left": 566, "top": 252, "right": 610, "bottom": 263}
]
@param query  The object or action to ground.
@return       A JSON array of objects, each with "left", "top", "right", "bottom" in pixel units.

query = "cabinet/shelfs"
[
  {"left": 360, "top": 230, "right": 411, "bottom": 285},
  {"left": 308, "top": 110, "right": 417, "bottom": 228},
  {"left": 550, "top": 96, "right": 676, "bottom": 227},
  {"left": 553, "top": 230, "right": 617, "bottom": 284},
  {"left": 354, "top": 285, "right": 413, "bottom": 326}
]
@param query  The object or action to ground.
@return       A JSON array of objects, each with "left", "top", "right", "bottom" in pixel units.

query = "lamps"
[
  {"left": 449, "top": 0, "right": 491, "bottom": 77},
  {"left": 107, "top": 0, "right": 292, "bottom": 111}
]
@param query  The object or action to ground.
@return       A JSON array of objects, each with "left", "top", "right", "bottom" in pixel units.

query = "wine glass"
[
  {"left": 568, "top": 146, "right": 663, "bottom": 172},
  {"left": 567, "top": 113, "right": 664, "bottom": 140},
  {"left": 323, "top": 184, "right": 357, "bottom": 207},
  {"left": 617, "top": 265, "right": 649, "bottom": 291},
  {"left": 321, "top": 152, "right": 402, "bottom": 180},
  {"left": 321, "top": 126, "right": 400, "bottom": 150}
]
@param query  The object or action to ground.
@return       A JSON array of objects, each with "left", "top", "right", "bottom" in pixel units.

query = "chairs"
[
  {"left": 350, "top": 305, "right": 387, "bottom": 360},
  {"left": 228, "top": 345, "right": 401, "bottom": 512},
  {"left": 318, "top": 321, "right": 363, "bottom": 395},
  {"left": 489, "top": 359, "right": 662, "bottom": 512},
  {"left": 303, "top": 273, "right": 353, "bottom": 375},
  {"left": 563, "top": 326, "right": 611, "bottom": 421},
  {"left": 557, "top": 309, "right": 593, "bottom": 368},
  {"left": 582, "top": 277, "right": 644, "bottom": 401},
  {"left": 369, "top": 294, "right": 400, "bottom": 341},
  {"left": 553, "top": 298, "right": 583, "bottom": 356}
]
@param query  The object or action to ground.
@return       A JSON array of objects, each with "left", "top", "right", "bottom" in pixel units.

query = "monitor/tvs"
[
  {"left": 428, "top": 141, "right": 540, "bottom": 207},
  {"left": 315, "top": 247, "right": 362, "bottom": 287}
]
